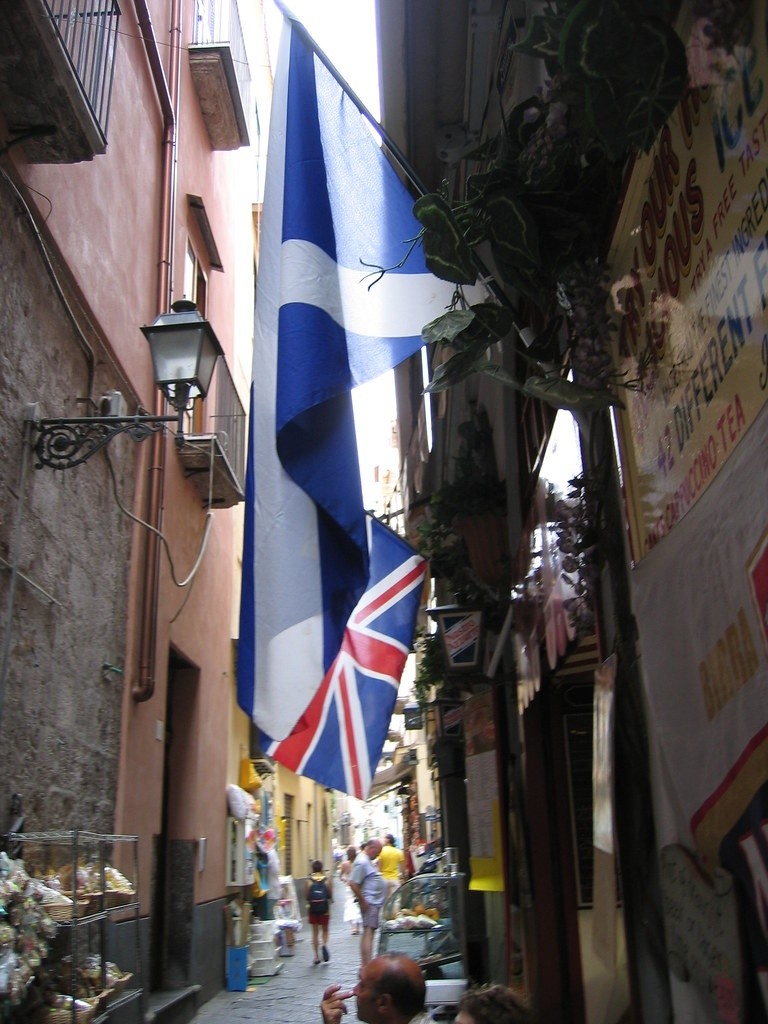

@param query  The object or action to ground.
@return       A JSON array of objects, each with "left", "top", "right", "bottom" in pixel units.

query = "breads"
[{"left": 393, "top": 906, "right": 439, "bottom": 921}]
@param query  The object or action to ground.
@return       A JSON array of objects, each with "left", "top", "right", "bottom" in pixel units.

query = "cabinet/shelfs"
[{"left": 0, "top": 828, "right": 151, "bottom": 1024}]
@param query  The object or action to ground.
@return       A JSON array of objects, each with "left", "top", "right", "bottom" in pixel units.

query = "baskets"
[
  {"left": 99, "top": 889, "right": 136, "bottom": 907},
  {"left": 40, "top": 897, "right": 90, "bottom": 921},
  {"left": 78, "top": 987, "right": 115, "bottom": 1013},
  {"left": 60, "top": 889, "right": 102, "bottom": 913},
  {"left": 37, "top": 1004, "right": 99, "bottom": 1024},
  {"left": 109, "top": 971, "right": 133, "bottom": 998}
]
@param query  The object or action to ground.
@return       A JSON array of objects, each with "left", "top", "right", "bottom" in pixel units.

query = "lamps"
[
  {"left": 424, "top": 593, "right": 488, "bottom": 673},
  {"left": 38, "top": 293, "right": 224, "bottom": 478},
  {"left": 434, "top": 695, "right": 465, "bottom": 739}
]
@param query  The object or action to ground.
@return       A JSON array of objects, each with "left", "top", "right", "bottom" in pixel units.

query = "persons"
[
  {"left": 377, "top": 833, "right": 410, "bottom": 922},
  {"left": 320, "top": 953, "right": 441, "bottom": 1024},
  {"left": 303, "top": 861, "right": 332, "bottom": 965},
  {"left": 348, "top": 838, "right": 387, "bottom": 965},
  {"left": 341, "top": 842, "right": 383, "bottom": 934},
  {"left": 454, "top": 981, "right": 549, "bottom": 1024}
]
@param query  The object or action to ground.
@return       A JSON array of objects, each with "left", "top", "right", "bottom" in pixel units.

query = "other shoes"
[
  {"left": 314, "top": 960, "right": 320, "bottom": 963},
  {"left": 322, "top": 946, "right": 329, "bottom": 962},
  {"left": 353, "top": 928, "right": 359, "bottom": 934}
]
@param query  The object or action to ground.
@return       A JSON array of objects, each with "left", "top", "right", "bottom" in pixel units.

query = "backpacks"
[{"left": 309, "top": 877, "right": 329, "bottom": 914}]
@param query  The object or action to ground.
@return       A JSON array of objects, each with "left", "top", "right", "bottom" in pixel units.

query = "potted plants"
[{"left": 432, "top": 406, "right": 504, "bottom": 583}]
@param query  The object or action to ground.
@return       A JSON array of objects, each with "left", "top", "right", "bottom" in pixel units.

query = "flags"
[
  {"left": 236, "top": 2, "right": 491, "bottom": 743},
  {"left": 258, "top": 504, "right": 430, "bottom": 799}
]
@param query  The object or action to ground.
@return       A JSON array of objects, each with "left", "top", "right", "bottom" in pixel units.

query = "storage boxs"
[{"left": 224, "top": 945, "right": 249, "bottom": 992}]
